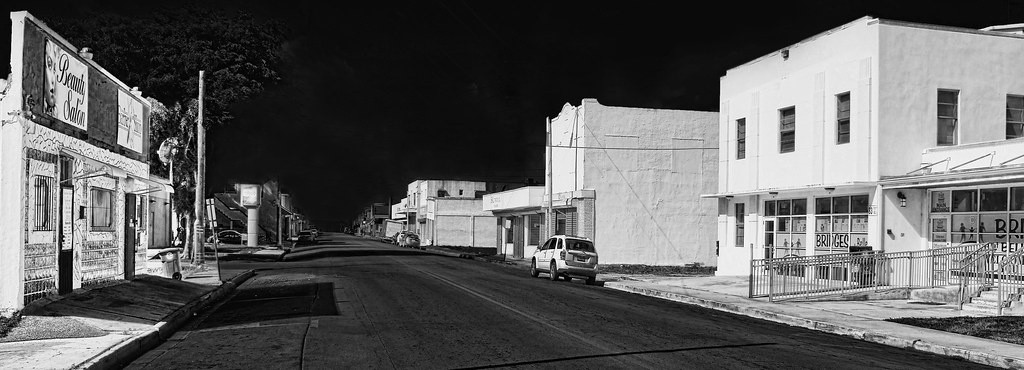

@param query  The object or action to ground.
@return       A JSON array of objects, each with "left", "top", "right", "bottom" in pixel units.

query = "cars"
[
  {"left": 207, "top": 230, "right": 247, "bottom": 245},
  {"left": 297, "top": 228, "right": 318, "bottom": 244},
  {"left": 392, "top": 229, "right": 421, "bottom": 249},
  {"left": 344, "top": 227, "right": 354, "bottom": 235}
]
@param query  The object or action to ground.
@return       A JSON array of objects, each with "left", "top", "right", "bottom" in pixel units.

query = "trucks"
[{"left": 379, "top": 219, "right": 404, "bottom": 243}]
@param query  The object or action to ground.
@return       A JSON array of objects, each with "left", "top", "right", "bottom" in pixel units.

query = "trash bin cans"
[
  {"left": 850, "top": 246, "right": 872, "bottom": 281},
  {"left": 158, "top": 249, "right": 183, "bottom": 281}
]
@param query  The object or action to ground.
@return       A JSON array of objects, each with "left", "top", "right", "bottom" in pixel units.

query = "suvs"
[{"left": 531, "top": 234, "right": 600, "bottom": 286}]
